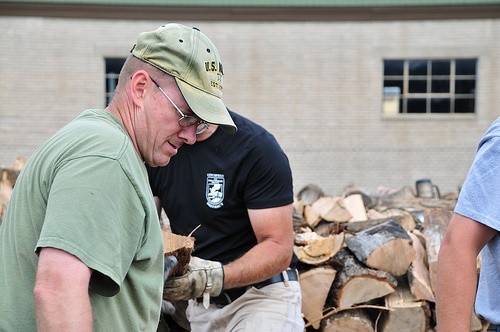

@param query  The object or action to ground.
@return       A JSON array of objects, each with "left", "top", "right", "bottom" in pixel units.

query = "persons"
[
  {"left": 435, "top": 117, "right": 500, "bottom": 332},
  {"left": 0, "top": 22, "right": 239, "bottom": 332},
  {"left": 145, "top": 107, "right": 306, "bottom": 332}
]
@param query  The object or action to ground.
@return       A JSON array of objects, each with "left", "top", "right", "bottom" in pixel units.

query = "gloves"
[
  {"left": 162, "top": 256, "right": 178, "bottom": 315},
  {"left": 163, "top": 254, "right": 224, "bottom": 309}
]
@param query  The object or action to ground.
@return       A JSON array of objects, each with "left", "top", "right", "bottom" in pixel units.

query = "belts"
[{"left": 196, "top": 270, "right": 298, "bottom": 309}]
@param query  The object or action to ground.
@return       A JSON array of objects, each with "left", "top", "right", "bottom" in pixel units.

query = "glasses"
[{"left": 130, "top": 75, "right": 209, "bottom": 134}]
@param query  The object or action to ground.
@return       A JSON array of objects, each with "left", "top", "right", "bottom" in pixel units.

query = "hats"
[{"left": 129, "top": 23, "right": 238, "bottom": 135}]
